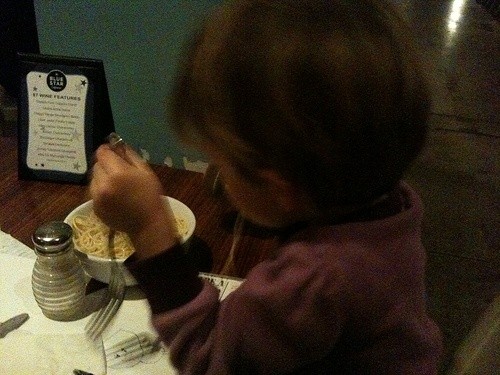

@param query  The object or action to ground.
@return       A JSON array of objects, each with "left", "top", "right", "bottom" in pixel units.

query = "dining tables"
[{"left": 0, "top": 134, "right": 281, "bottom": 375}]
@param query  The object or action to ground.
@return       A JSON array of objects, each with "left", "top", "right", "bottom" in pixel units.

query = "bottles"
[{"left": 30, "top": 220, "right": 86, "bottom": 322}]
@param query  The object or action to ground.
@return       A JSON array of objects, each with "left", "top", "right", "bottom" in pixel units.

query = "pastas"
[{"left": 73, "top": 205, "right": 188, "bottom": 258}]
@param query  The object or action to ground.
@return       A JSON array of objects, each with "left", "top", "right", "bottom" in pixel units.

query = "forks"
[{"left": 83, "top": 133, "right": 128, "bottom": 345}]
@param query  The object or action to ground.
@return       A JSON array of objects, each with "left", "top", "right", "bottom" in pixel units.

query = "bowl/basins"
[{"left": 63, "top": 193, "right": 198, "bottom": 287}]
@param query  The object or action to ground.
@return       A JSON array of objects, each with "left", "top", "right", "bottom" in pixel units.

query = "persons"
[{"left": 88, "top": 1, "right": 455, "bottom": 375}]
